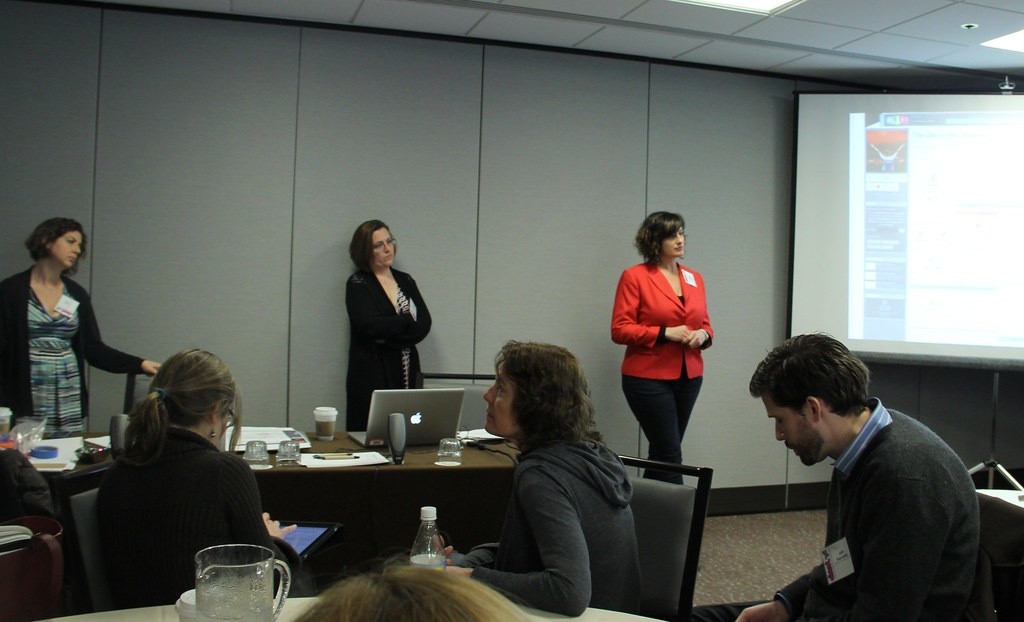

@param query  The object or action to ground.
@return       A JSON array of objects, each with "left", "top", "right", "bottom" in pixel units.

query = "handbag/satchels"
[{"left": 0, "top": 515, "right": 64, "bottom": 622}]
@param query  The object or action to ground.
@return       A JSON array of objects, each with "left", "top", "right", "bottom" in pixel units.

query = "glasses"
[
  {"left": 373, "top": 238, "right": 397, "bottom": 250},
  {"left": 225, "top": 408, "right": 238, "bottom": 427}
]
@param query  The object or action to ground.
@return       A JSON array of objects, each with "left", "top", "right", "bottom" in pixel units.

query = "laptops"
[{"left": 346, "top": 388, "right": 466, "bottom": 448}]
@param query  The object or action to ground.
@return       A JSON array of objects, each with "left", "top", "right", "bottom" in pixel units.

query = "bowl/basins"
[{"left": 74, "top": 446, "right": 108, "bottom": 465}]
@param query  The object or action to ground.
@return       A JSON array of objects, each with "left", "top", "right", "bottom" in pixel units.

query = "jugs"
[{"left": 193, "top": 543, "right": 291, "bottom": 622}]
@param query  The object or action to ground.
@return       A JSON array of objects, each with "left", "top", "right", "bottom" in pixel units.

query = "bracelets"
[{"left": 699, "top": 329, "right": 709, "bottom": 341}]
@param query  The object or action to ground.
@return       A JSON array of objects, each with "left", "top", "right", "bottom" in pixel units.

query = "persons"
[
  {"left": 94, "top": 348, "right": 320, "bottom": 611},
  {"left": 435, "top": 341, "right": 642, "bottom": 617},
  {"left": 292, "top": 558, "right": 532, "bottom": 622},
  {"left": 693, "top": 333, "right": 978, "bottom": 622},
  {"left": 610, "top": 211, "right": 714, "bottom": 485},
  {"left": 0, "top": 217, "right": 160, "bottom": 432},
  {"left": 344, "top": 219, "right": 432, "bottom": 432}
]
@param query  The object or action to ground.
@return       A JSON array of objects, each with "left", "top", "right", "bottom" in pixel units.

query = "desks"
[
  {"left": 26, "top": 432, "right": 522, "bottom": 574},
  {"left": 34, "top": 594, "right": 667, "bottom": 622}
]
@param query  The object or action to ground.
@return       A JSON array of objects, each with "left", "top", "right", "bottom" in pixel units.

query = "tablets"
[{"left": 275, "top": 519, "right": 340, "bottom": 559}]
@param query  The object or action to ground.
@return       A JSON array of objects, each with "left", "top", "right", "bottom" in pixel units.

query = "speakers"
[
  {"left": 109, "top": 414, "right": 131, "bottom": 460},
  {"left": 388, "top": 413, "right": 406, "bottom": 465}
]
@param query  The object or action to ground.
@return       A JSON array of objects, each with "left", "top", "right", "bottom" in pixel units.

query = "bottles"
[{"left": 408, "top": 505, "right": 447, "bottom": 574}]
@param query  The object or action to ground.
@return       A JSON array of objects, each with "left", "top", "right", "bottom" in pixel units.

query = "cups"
[
  {"left": 0, "top": 407, "right": 13, "bottom": 433},
  {"left": 437, "top": 438, "right": 463, "bottom": 466},
  {"left": 313, "top": 406, "right": 338, "bottom": 441},
  {"left": 242, "top": 441, "right": 270, "bottom": 470},
  {"left": 276, "top": 440, "right": 301, "bottom": 470},
  {"left": 175, "top": 589, "right": 197, "bottom": 622}
]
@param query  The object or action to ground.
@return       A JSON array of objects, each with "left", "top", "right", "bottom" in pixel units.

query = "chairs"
[
  {"left": 977, "top": 492, "right": 1024, "bottom": 622},
  {"left": 50, "top": 460, "right": 117, "bottom": 616},
  {"left": 619, "top": 454, "right": 714, "bottom": 622}
]
